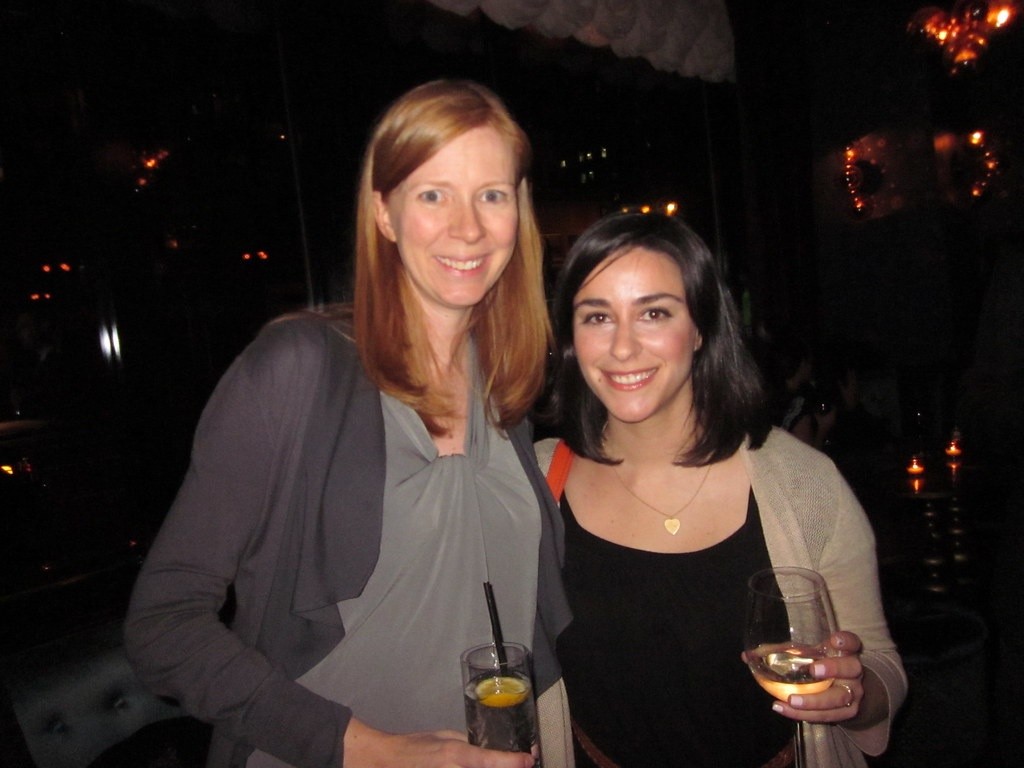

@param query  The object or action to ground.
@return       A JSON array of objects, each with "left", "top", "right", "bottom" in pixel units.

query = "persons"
[
  {"left": 123, "top": 74, "right": 585, "bottom": 768},
  {"left": 534, "top": 211, "right": 907, "bottom": 767},
  {"left": 0, "top": 306, "right": 86, "bottom": 418},
  {"left": 769, "top": 335, "right": 838, "bottom": 452}
]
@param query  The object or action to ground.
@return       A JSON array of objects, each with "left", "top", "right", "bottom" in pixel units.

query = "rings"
[{"left": 838, "top": 684, "right": 854, "bottom": 706}]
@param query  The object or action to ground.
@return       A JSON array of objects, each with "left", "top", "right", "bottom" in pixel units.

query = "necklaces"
[{"left": 599, "top": 419, "right": 711, "bottom": 536}]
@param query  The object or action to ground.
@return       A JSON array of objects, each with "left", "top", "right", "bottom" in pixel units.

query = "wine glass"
[{"left": 741, "top": 567, "right": 841, "bottom": 768}]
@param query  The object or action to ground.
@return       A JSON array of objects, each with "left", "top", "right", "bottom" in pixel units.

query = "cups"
[{"left": 460, "top": 643, "right": 541, "bottom": 768}]
